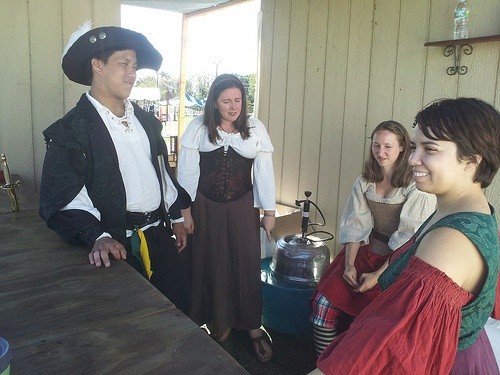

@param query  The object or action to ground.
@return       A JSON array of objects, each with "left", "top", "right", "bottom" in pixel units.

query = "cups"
[{"left": 0, "top": 337, "right": 12, "bottom": 375}]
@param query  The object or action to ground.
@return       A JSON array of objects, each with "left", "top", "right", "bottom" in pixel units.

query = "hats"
[{"left": 61, "top": 26, "right": 162, "bottom": 85}]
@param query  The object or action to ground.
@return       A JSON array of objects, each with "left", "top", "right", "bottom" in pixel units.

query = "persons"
[
  {"left": 308, "top": 97, "right": 500, "bottom": 375},
  {"left": 38, "top": 20, "right": 192, "bottom": 320},
  {"left": 312, "top": 120, "right": 437, "bottom": 360},
  {"left": 178, "top": 72, "right": 276, "bottom": 362}
]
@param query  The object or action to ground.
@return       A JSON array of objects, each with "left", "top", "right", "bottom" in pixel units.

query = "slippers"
[
  {"left": 250, "top": 331, "right": 274, "bottom": 363},
  {"left": 212, "top": 327, "right": 233, "bottom": 342}
]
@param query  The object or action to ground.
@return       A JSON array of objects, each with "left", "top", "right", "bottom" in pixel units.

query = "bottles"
[{"left": 454, "top": 0, "right": 468, "bottom": 38}]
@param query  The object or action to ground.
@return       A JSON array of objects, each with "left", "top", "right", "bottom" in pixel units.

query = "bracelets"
[{"left": 264, "top": 214, "right": 275, "bottom": 217}]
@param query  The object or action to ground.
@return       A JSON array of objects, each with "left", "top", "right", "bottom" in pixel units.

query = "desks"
[{"left": 0, "top": 174, "right": 251, "bottom": 375}]
